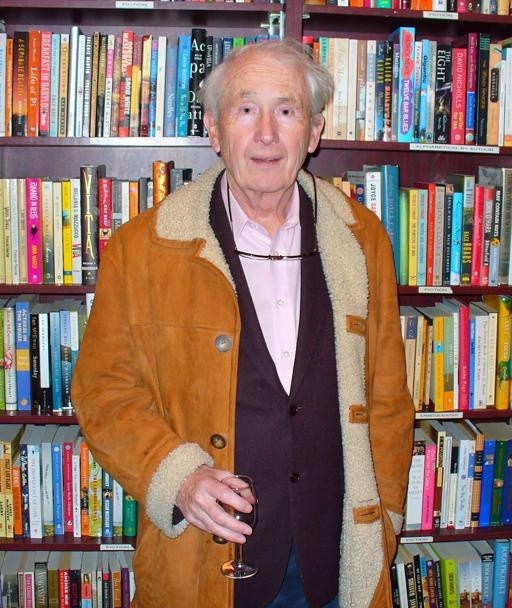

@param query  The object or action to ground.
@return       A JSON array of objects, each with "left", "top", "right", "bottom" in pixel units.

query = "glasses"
[{"left": 226, "top": 169, "right": 318, "bottom": 260}]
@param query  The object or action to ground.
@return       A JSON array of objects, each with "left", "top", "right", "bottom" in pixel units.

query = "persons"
[{"left": 70, "top": 39, "right": 416, "bottom": 607}]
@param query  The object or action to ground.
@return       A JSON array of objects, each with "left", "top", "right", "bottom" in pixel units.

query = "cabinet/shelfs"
[{"left": 1, "top": 0, "right": 512, "bottom": 608}]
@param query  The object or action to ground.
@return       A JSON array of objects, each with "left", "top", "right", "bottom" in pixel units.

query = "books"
[
  {"left": 209, "top": 0, "right": 286, "bottom": 4},
  {"left": 390, "top": 422, "right": 512, "bottom": 607},
  {"left": 0, "top": 424, "right": 136, "bottom": 538},
  {"left": 1, "top": 19, "right": 280, "bottom": 138},
  {"left": 329, "top": 164, "right": 511, "bottom": 411},
  {"left": 1, "top": 293, "right": 96, "bottom": 416},
  {"left": 302, "top": 1, "right": 512, "bottom": 147},
  {"left": 0, "top": 551, "right": 135, "bottom": 607},
  {"left": 1, "top": 161, "right": 193, "bottom": 284}
]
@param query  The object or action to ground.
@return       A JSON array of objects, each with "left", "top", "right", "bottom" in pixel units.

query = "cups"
[{"left": 217, "top": 474, "right": 259, "bottom": 581}]
